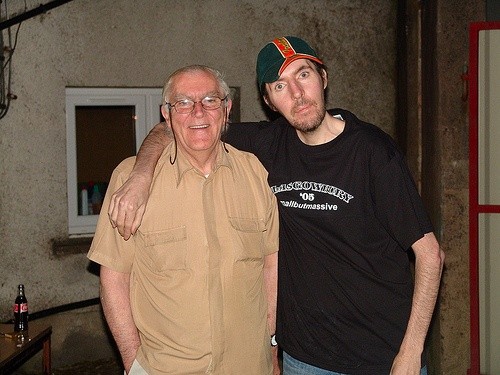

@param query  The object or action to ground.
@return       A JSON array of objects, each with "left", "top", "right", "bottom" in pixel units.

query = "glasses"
[{"left": 165, "top": 94, "right": 228, "bottom": 114}]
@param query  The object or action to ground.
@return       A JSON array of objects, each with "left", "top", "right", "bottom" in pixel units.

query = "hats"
[{"left": 257, "top": 33, "right": 326, "bottom": 94}]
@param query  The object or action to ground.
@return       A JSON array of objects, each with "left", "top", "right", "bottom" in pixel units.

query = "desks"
[{"left": 0, "top": 324, "right": 53, "bottom": 375}]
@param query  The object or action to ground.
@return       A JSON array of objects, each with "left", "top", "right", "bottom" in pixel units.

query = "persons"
[
  {"left": 87, "top": 65, "right": 283, "bottom": 375},
  {"left": 108, "top": 36, "right": 446, "bottom": 375}
]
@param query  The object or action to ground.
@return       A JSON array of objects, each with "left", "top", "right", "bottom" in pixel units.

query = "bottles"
[
  {"left": 14, "top": 284, "right": 29, "bottom": 332},
  {"left": 79, "top": 184, "right": 102, "bottom": 215}
]
